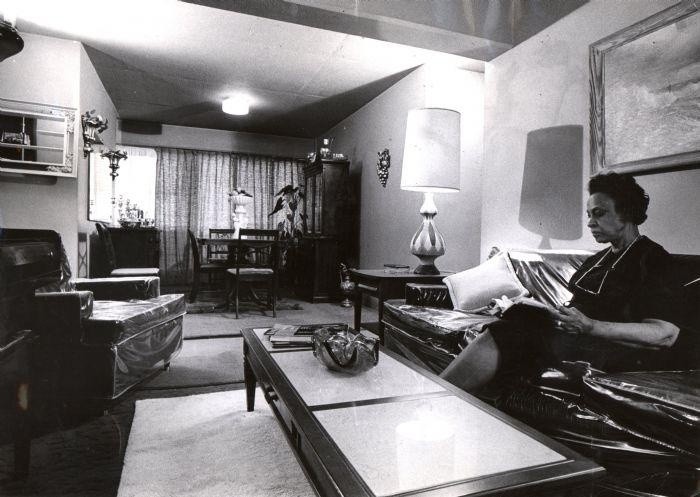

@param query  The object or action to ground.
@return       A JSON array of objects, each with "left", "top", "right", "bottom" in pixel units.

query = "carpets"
[{"left": 115, "top": 384, "right": 322, "bottom": 497}]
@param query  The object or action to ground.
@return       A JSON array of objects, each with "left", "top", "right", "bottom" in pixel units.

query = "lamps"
[
  {"left": 399, "top": 107, "right": 462, "bottom": 275},
  {"left": 221, "top": 98, "right": 249, "bottom": 116}
]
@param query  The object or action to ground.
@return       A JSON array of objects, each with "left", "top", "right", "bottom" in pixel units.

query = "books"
[
  {"left": 264, "top": 322, "right": 348, "bottom": 349},
  {"left": 487, "top": 296, "right": 568, "bottom": 328}
]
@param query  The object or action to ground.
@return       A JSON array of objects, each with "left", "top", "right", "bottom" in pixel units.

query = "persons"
[{"left": 439, "top": 171, "right": 682, "bottom": 397}]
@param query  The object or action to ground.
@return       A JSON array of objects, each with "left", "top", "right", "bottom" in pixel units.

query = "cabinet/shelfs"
[
  {"left": 0, "top": 98, "right": 80, "bottom": 178},
  {"left": 298, "top": 160, "right": 350, "bottom": 304}
]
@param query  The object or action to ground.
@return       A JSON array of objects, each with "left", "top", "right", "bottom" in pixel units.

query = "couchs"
[{"left": 380, "top": 247, "right": 700, "bottom": 497}]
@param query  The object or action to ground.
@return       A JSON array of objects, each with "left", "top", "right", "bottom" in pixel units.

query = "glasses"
[{"left": 575, "top": 265, "right": 614, "bottom": 297}]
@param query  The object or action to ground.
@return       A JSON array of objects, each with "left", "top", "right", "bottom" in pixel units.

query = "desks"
[
  {"left": 197, "top": 237, "right": 303, "bottom": 309},
  {"left": 351, "top": 269, "right": 454, "bottom": 347},
  {"left": 104, "top": 227, "right": 162, "bottom": 266}
]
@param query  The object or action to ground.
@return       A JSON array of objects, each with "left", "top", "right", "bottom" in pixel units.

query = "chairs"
[
  {"left": 207, "top": 228, "right": 236, "bottom": 262},
  {"left": 188, "top": 229, "right": 236, "bottom": 303},
  {"left": 225, "top": 228, "right": 279, "bottom": 320},
  {"left": 0, "top": 269, "right": 62, "bottom": 483},
  {"left": 95, "top": 221, "right": 160, "bottom": 276},
  {"left": 0, "top": 229, "right": 185, "bottom": 414}
]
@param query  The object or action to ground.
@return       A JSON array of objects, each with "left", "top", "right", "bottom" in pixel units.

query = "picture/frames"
[{"left": 589, "top": 0, "right": 700, "bottom": 178}]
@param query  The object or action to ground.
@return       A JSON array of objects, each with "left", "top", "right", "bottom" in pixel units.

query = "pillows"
[{"left": 443, "top": 251, "right": 530, "bottom": 312}]
